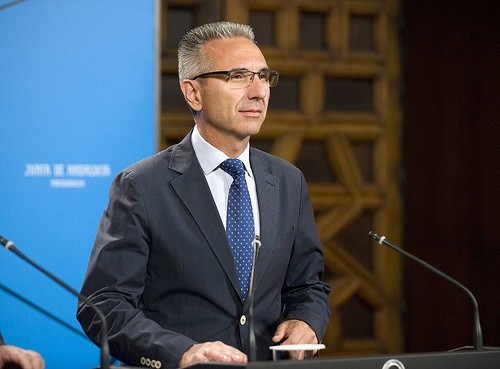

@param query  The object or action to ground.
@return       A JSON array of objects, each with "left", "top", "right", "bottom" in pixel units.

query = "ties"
[{"left": 218, "top": 157, "right": 255, "bottom": 307}]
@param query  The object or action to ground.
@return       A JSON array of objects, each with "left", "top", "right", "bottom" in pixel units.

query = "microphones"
[
  {"left": 247, "top": 235, "right": 261, "bottom": 363},
  {"left": 368, "top": 231, "right": 483, "bottom": 351},
  {"left": 0, "top": 235, "right": 110, "bottom": 369}
]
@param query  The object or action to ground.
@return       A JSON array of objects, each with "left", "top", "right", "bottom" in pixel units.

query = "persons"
[
  {"left": 74, "top": 23, "right": 330, "bottom": 369},
  {"left": 0, "top": 331, "right": 45, "bottom": 369}
]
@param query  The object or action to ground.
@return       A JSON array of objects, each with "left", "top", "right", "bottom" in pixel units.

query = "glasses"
[{"left": 193, "top": 68, "right": 279, "bottom": 88}]
